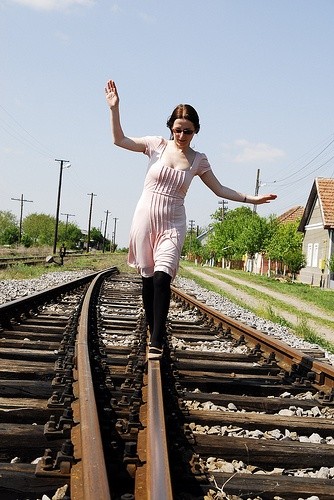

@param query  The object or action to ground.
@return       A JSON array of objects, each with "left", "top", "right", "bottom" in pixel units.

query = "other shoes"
[
  {"left": 147, "top": 346, "right": 164, "bottom": 359},
  {"left": 148, "top": 341, "right": 153, "bottom": 348}
]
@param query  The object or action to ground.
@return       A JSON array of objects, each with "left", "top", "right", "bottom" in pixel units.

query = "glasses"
[{"left": 172, "top": 129, "right": 194, "bottom": 134}]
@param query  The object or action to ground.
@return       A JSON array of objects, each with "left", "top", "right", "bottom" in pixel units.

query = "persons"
[{"left": 105, "top": 81, "right": 278, "bottom": 359}]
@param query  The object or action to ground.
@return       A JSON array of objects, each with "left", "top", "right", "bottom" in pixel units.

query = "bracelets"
[{"left": 244, "top": 197, "right": 246, "bottom": 202}]
